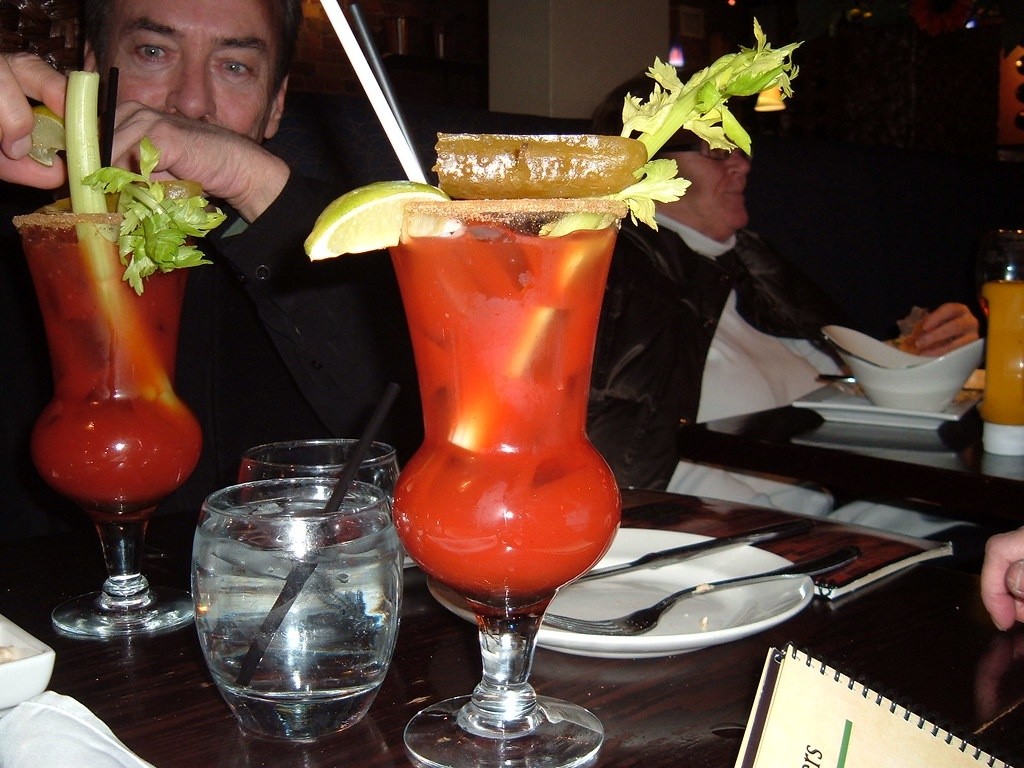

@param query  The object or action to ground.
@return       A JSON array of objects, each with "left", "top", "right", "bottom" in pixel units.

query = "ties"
[{"left": 716, "top": 246, "right": 825, "bottom": 339}]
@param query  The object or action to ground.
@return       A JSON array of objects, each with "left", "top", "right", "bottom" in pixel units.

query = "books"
[
  {"left": 618, "top": 488, "right": 954, "bottom": 599},
  {"left": 733, "top": 641, "right": 1015, "bottom": 768}
]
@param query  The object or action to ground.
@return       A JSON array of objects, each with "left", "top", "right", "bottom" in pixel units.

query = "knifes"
[{"left": 569, "top": 518, "right": 816, "bottom": 582}]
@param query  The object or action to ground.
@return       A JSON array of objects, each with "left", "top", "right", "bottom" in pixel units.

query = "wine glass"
[
  {"left": 393, "top": 230, "right": 622, "bottom": 768},
  {"left": 20, "top": 235, "right": 202, "bottom": 640}
]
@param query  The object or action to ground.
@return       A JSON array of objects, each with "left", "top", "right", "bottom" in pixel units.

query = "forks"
[{"left": 543, "top": 544, "right": 863, "bottom": 636}]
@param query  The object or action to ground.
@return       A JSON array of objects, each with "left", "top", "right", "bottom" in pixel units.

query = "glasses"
[{"left": 652, "top": 130, "right": 727, "bottom": 159}]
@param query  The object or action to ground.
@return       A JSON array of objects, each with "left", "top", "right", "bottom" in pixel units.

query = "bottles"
[{"left": 982, "top": 264, "right": 1024, "bottom": 456}]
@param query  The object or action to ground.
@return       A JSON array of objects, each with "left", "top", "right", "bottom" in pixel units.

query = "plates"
[
  {"left": 791, "top": 369, "right": 987, "bottom": 429},
  {"left": 426, "top": 529, "right": 815, "bottom": 658},
  {"left": 791, "top": 422, "right": 975, "bottom": 474}
]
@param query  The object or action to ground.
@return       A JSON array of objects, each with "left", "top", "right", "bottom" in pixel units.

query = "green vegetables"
[
  {"left": 84, "top": 137, "right": 228, "bottom": 296},
  {"left": 535, "top": 15, "right": 806, "bottom": 239}
]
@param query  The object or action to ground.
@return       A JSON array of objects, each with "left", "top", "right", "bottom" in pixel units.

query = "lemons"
[
  {"left": 304, "top": 179, "right": 462, "bottom": 261},
  {"left": 27, "top": 105, "right": 65, "bottom": 166}
]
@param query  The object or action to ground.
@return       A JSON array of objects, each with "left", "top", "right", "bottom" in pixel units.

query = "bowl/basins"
[{"left": 822, "top": 324, "right": 984, "bottom": 412}]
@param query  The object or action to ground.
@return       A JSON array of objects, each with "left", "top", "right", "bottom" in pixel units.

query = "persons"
[
  {"left": 587, "top": 68, "right": 979, "bottom": 420},
  {"left": 0, "top": 1, "right": 423, "bottom": 521}
]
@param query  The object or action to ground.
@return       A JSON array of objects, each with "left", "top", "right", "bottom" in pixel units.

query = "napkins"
[{"left": 0, "top": 691, "right": 157, "bottom": 768}]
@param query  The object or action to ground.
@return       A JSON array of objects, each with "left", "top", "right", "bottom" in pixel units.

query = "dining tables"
[
  {"left": 0, "top": 483, "right": 1024, "bottom": 768},
  {"left": 678, "top": 379, "right": 1024, "bottom": 527}
]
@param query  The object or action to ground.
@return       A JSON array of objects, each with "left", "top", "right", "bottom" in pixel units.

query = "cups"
[
  {"left": 239, "top": 440, "right": 399, "bottom": 503},
  {"left": 190, "top": 477, "right": 402, "bottom": 740}
]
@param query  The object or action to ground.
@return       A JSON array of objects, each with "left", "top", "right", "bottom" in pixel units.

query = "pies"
[{"left": 893, "top": 307, "right": 933, "bottom": 355}]
